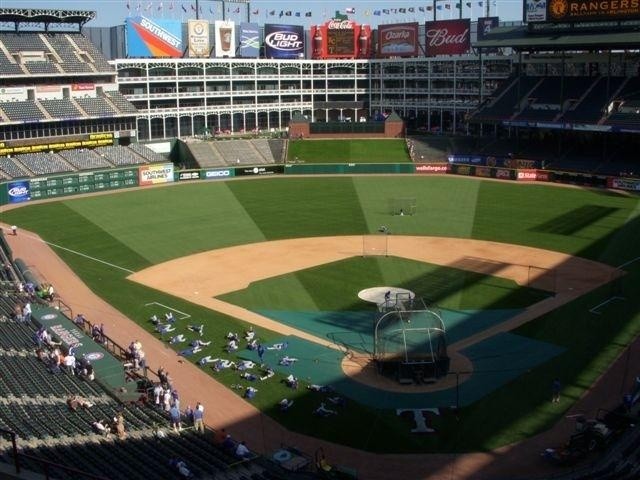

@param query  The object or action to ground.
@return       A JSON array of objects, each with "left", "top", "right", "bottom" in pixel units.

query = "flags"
[
  {"left": 117, "top": 0, "right": 313, "bottom": 20},
  {"left": 345, "top": 7, "right": 355, "bottom": 14},
  {"left": 362, "top": 0, "right": 497, "bottom": 17}
]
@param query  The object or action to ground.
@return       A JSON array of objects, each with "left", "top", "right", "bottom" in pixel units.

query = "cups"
[{"left": 218, "top": 26, "right": 232, "bottom": 50}]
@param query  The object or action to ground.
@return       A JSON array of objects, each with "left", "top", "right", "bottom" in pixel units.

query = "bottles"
[
  {"left": 357, "top": 24, "right": 369, "bottom": 58},
  {"left": 312, "top": 24, "right": 323, "bottom": 56}
]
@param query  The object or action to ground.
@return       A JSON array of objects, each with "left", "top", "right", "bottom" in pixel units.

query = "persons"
[
  {"left": 384, "top": 290, "right": 392, "bottom": 308},
  {"left": 574, "top": 410, "right": 583, "bottom": 432},
  {"left": 12, "top": 278, "right": 253, "bottom": 478},
  {"left": 10, "top": 223, "right": 18, "bottom": 235},
  {"left": 621, "top": 374, "right": 640, "bottom": 414},
  {"left": 545, "top": 375, "right": 566, "bottom": 406},
  {"left": 536, "top": 446, "right": 559, "bottom": 459},
  {"left": 399, "top": 207, "right": 403, "bottom": 215},
  {"left": 146, "top": 310, "right": 349, "bottom": 418}
]
